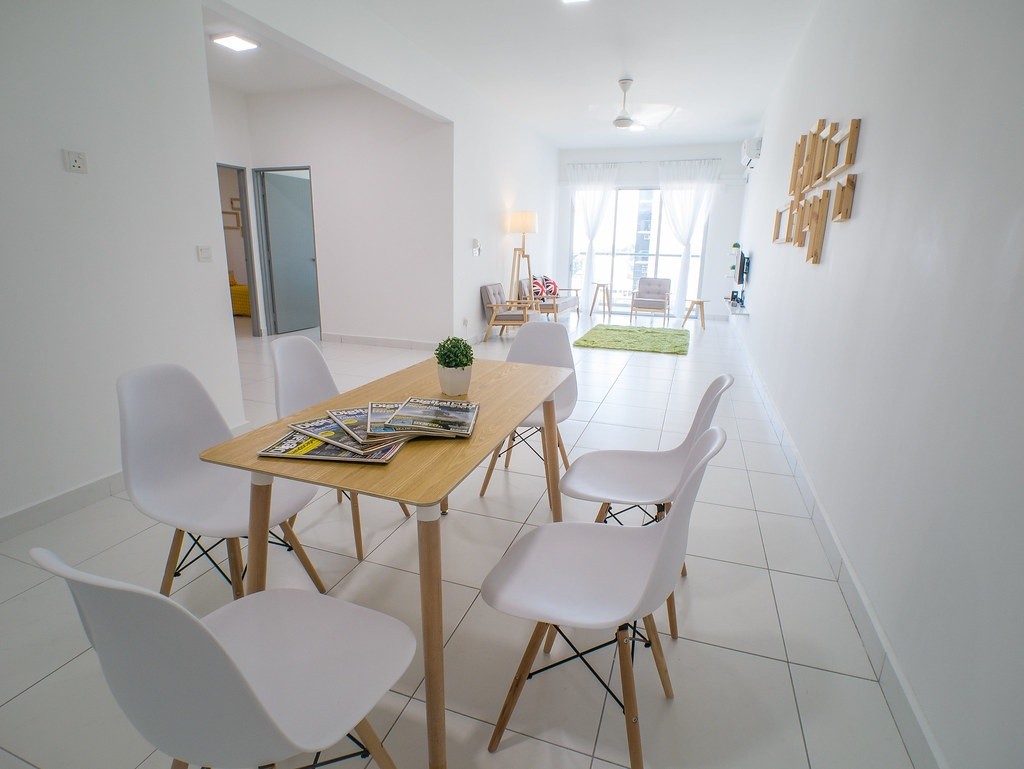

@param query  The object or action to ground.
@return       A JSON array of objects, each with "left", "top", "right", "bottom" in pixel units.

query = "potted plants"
[{"left": 433, "top": 336, "right": 477, "bottom": 398}]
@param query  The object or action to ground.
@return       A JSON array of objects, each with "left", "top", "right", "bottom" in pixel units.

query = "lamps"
[{"left": 505, "top": 209, "right": 539, "bottom": 309}]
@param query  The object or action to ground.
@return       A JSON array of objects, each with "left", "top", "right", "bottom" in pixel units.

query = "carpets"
[{"left": 572, "top": 323, "right": 692, "bottom": 357}]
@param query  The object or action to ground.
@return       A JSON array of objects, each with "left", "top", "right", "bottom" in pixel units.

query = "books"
[{"left": 258, "top": 396, "right": 480, "bottom": 463}]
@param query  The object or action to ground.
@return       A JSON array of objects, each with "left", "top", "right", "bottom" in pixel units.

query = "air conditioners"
[
  {"left": 593, "top": 79, "right": 654, "bottom": 130},
  {"left": 740, "top": 136, "right": 763, "bottom": 170}
]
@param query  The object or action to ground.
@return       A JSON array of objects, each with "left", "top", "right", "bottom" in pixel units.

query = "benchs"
[
  {"left": 680, "top": 298, "right": 712, "bottom": 330},
  {"left": 518, "top": 276, "right": 580, "bottom": 321}
]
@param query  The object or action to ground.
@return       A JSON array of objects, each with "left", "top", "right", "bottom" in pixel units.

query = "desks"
[
  {"left": 199, "top": 354, "right": 572, "bottom": 769},
  {"left": 589, "top": 282, "right": 613, "bottom": 319}
]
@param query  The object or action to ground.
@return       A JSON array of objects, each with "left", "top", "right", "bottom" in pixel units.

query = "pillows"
[
  {"left": 542, "top": 274, "right": 561, "bottom": 298},
  {"left": 532, "top": 274, "right": 546, "bottom": 302}
]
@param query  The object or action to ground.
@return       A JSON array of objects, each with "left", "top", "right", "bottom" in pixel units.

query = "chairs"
[
  {"left": 270, "top": 334, "right": 411, "bottom": 563},
  {"left": 556, "top": 372, "right": 736, "bottom": 640},
  {"left": 479, "top": 282, "right": 542, "bottom": 343},
  {"left": 477, "top": 322, "right": 578, "bottom": 512},
  {"left": 627, "top": 277, "right": 673, "bottom": 326},
  {"left": 28, "top": 545, "right": 419, "bottom": 768},
  {"left": 479, "top": 426, "right": 727, "bottom": 768},
  {"left": 114, "top": 363, "right": 327, "bottom": 607}
]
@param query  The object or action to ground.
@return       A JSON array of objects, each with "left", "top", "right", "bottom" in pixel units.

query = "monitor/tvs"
[{"left": 734, "top": 250, "right": 745, "bottom": 285}]
